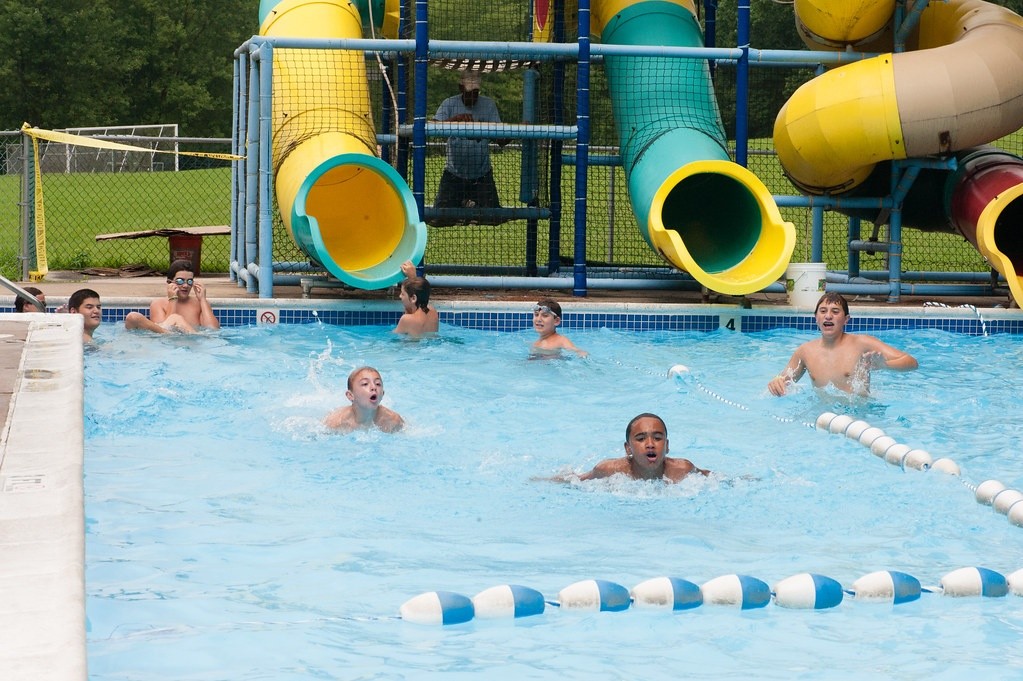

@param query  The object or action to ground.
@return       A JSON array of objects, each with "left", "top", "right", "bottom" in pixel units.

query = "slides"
[
  {"left": 772, "top": 0, "right": 1023, "bottom": 316},
  {"left": 594, "top": 0, "right": 797, "bottom": 296},
  {"left": 258, "top": 0, "right": 429, "bottom": 292}
]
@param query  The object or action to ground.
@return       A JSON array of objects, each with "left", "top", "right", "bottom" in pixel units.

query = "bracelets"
[{"left": 168, "top": 296, "right": 179, "bottom": 301}]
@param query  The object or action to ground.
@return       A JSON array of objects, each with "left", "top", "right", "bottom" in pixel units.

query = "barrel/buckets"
[{"left": 786, "top": 262, "right": 827, "bottom": 306}]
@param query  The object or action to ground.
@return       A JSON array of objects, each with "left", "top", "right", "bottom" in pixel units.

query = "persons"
[
  {"left": 527, "top": 413, "right": 762, "bottom": 493},
  {"left": 150, "top": 259, "right": 220, "bottom": 333},
  {"left": 14, "top": 287, "right": 207, "bottom": 359},
  {"left": 767, "top": 290, "right": 918, "bottom": 408},
  {"left": 527, "top": 299, "right": 589, "bottom": 362},
  {"left": 319, "top": 366, "right": 406, "bottom": 437},
  {"left": 432, "top": 70, "right": 529, "bottom": 208},
  {"left": 392, "top": 260, "right": 438, "bottom": 339}
]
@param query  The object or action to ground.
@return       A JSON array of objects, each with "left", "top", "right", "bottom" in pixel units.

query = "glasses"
[
  {"left": 41, "top": 300, "right": 47, "bottom": 307},
  {"left": 175, "top": 278, "right": 193, "bottom": 286},
  {"left": 533, "top": 305, "right": 551, "bottom": 314}
]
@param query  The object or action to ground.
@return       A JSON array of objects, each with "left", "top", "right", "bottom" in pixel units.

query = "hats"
[{"left": 459, "top": 70, "right": 481, "bottom": 91}]
